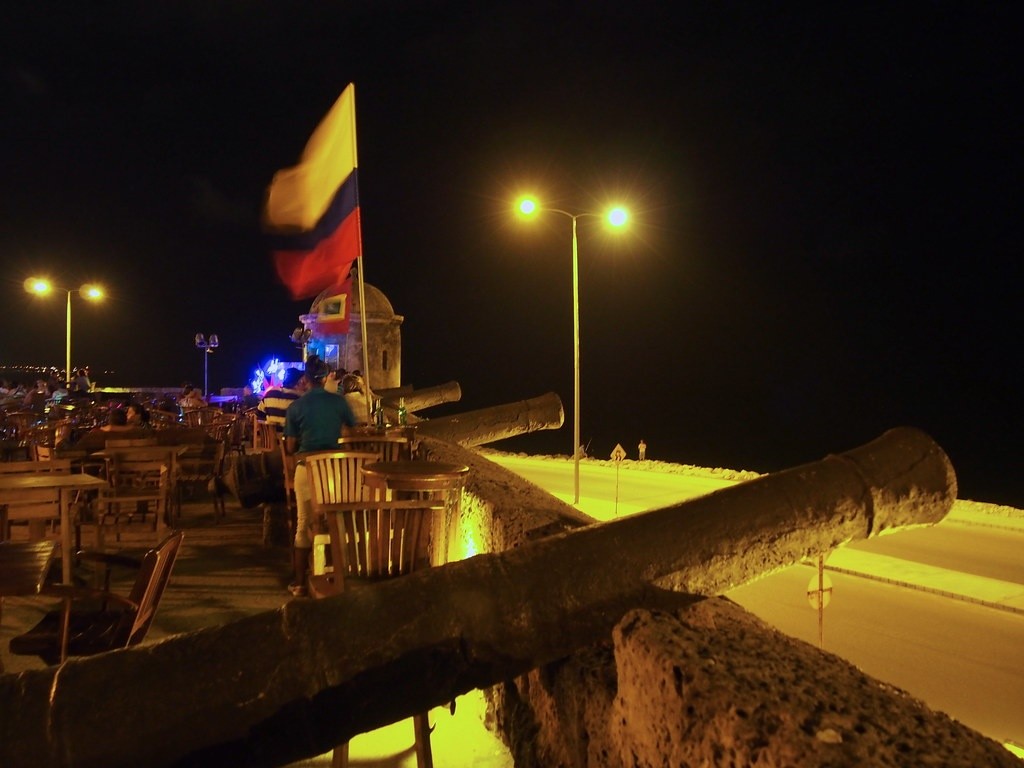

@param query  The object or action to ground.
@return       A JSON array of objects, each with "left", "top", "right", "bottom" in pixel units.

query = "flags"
[{"left": 266, "top": 83, "right": 362, "bottom": 299}]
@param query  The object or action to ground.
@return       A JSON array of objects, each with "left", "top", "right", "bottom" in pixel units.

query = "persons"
[
  {"left": 255, "top": 365, "right": 367, "bottom": 445},
  {"left": 638, "top": 440, "right": 647, "bottom": 459},
  {"left": 283, "top": 355, "right": 354, "bottom": 596},
  {"left": 0, "top": 369, "right": 209, "bottom": 450}
]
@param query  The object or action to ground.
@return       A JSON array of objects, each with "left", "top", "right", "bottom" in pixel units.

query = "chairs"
[{"left": 0, "top": 416, "right": 444, "bottom": 768}]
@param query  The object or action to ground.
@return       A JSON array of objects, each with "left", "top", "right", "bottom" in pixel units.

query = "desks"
[
  {"left": 342, "top": 424, "right": 415, "bottom": 461},
  {"left": 90, "top": 446, "right": 188, "bottom": 533},
  {"left": 0, "top": 472, "right": 110, "bottom": 585},
  {"left": 361, "top": 460, "right": 470, "bottom": 567}
]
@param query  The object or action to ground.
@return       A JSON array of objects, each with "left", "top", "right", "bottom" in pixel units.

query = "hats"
[
  {"left": 305, "top": 355, "right": 329, "bottom": 379},
  {"left": 280, "top": 367, "right": 304, "bottom": 388}
]
